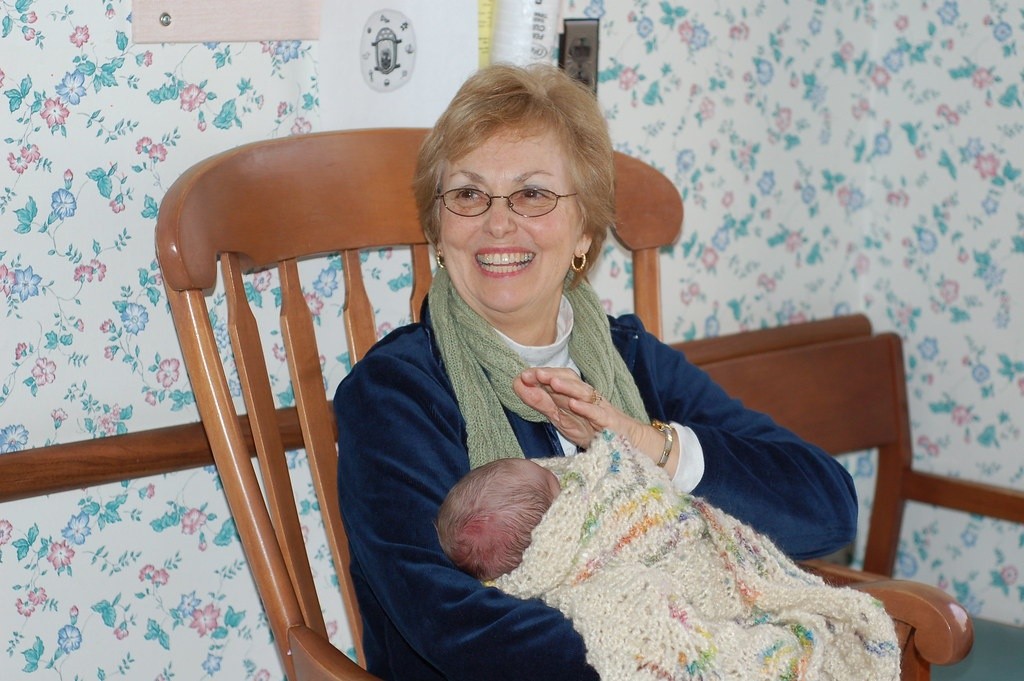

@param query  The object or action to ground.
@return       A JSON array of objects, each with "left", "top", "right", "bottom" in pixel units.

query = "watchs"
[{"left": 651, "top": 419, "right": 674, "bottom": 467}]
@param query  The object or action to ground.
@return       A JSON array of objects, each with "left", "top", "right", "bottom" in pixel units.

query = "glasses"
[{"left": 435, "top": 187, "right": 578, "bottom": 219}]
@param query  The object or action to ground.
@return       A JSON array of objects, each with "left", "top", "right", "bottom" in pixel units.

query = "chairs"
[
  {"left": 666, "top": 315, "right": 1024, "bottom": 681},
  {"left": 156, "top": 129, "right": 974, "bottom": 681}
]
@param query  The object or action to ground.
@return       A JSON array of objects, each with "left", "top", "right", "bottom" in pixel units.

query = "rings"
[{"left": 588, "top": 390, "right": 599, "bottom": 404}]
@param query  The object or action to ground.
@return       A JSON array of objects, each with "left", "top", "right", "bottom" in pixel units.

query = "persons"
[
  {"left": 334, "top": 65, "right": 861, "bottom": 681},
  {"left": 435, "top": 428, "right": 900, "bottom": 681}
]
